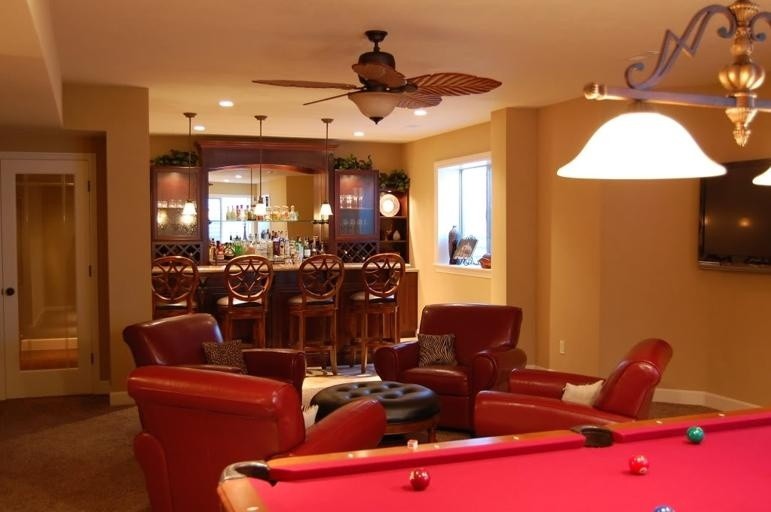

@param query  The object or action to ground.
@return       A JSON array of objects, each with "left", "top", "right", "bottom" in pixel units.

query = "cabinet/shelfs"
[{"left": 149, "top": 136, "right": 410, "bottom": 262}]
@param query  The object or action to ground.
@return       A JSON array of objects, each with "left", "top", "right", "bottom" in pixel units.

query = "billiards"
[
  {"left": 629, "top": 451, "right": 651, "bottom": 476},
  {"left": 686, "top": 425, "right": 706, "bottom": 445},
  {"left": 653, "top": 502, "right": 675, "bottom": 512},
  {"left": 408, "top": 466, "right": 431, "bottom": 490}
]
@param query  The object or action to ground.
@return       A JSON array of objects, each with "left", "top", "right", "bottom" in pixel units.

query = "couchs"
[
  {"left": 473, "top": 338, "right": 674, "bottom": 438},
  {"left": 372, "top": 302, "right": 528, "bottom": 433},
  {"left": 121, "top": 313, "right": 307, "bottom": 429},
  {"left": 126, "top": 364, "right": 387, "bottom": 512}
]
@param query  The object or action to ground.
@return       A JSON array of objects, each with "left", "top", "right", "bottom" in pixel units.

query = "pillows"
[
  {"left": 302, "top": 405, "right": 318, "bottom": 429},
  {"left": 202, "top": 339, "right": 248, "bottom": 375},
  {"left": 418, "top": 333, "right": 456, "bottom": 366},
  {"left": 562, "top": 379, "right": 605, "bottom": 406}
]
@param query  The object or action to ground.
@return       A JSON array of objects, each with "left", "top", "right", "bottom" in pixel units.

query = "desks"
[
  {"left": 211, "top": 405, "right": 771, "bottom": 512},
  {"left": 149, "top": 264, "right": 418, "bottom": 361}
]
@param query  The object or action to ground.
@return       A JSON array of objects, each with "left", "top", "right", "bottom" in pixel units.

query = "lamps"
[
  {"left": 552, "top": 0, "right": 770, "bottom": 191},
  {"left": 345, "top": 90, "right": 405, "bottom": 126},
  {"left": 182, "top": 110, "right": 336, "bottom": 218}
]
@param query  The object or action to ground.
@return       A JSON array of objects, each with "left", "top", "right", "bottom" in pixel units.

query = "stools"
[
  {"left": 215, "top": 255, "right": 274, "bottom": 351},
  {"left": 286, "top": 252, "right": 345, "bottom": 376},
  {"left": 347, "top": 253, "right": 405, "bottom": 374},
  {"left": 151, "top": 257, "right": 201, "bottom": 320},
  {"left": 310, "top": 380, "right": 442, "bottom": 448}
]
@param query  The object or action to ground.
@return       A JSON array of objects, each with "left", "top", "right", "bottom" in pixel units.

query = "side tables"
[{"left": 301, "top": 365, "right": 382, "bottom": 410}]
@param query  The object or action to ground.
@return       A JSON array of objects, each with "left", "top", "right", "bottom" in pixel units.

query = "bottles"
[
  {"left": 227, "top": 204, "right": 298, "bottom": 220},
  {"left": 393, "top": 229, "right": 401, "bottom": 241},
  {"left": 209, "top": 230, "right": 326, "bottom": 267},
  {"left": 449, "top": 225, "right": 462, "bottom": 258}
]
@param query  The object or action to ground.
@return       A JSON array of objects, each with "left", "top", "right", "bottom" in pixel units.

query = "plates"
[{"left": 380, "top": 194, "right": 400, "bottom": 217}]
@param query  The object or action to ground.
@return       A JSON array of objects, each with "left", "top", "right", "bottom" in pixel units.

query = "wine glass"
[
  {"left": 352, "top": 187, "right": 366, "bottom": 209},
  {"left": 384, "top": 224, "right": 393, "bottom": 241}
]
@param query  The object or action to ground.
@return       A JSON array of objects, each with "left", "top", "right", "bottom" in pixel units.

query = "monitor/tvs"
[{"left": 696, "top": 157, "right": 771, "bottom": 273}]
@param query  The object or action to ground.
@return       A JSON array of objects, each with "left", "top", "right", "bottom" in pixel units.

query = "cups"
[
  {"left": 343, "top": 219, "right": 369, "bottom": 234},
  {"left": 340, "top": 195, "right": 353, "bottom": 209},
  {"left": 158, "top": 199, "right": 198, "bottom": 208},
  {"left": 157, "top": 214, "right": 197, "bottom": 234}
]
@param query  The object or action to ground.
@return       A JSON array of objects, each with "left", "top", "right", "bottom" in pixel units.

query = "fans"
[{"left": 251, "top": 28, "right": 506, "bottom": 111}]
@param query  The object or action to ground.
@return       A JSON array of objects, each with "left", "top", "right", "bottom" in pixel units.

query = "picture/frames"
[{"left": 453, "top": 238, "right": 478, "bottom": 259}]
